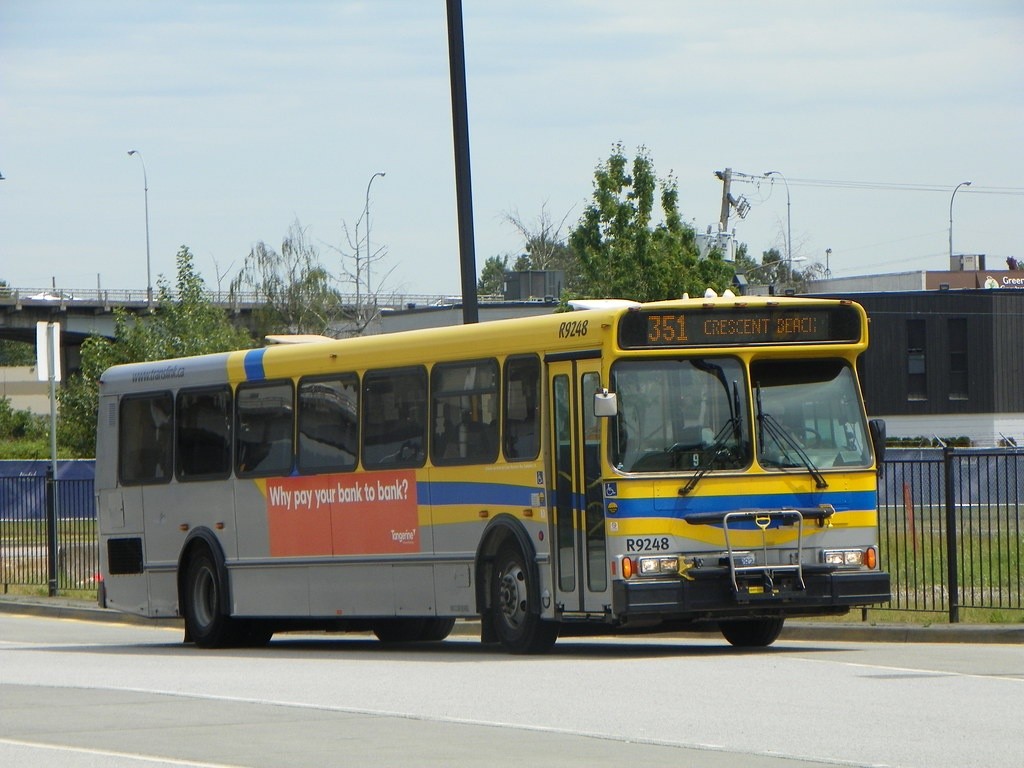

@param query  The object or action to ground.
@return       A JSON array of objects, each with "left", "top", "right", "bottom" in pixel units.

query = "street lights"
[
  {"left": 366, "top": 172, "right": 389, "bottom": 307},
  {"left": 126, "top": 149, "right": 153, "bottom": 313},
  {"left": 764, "top": 171, "right": 791, "bottom": 286},
  {"left": 949, "top": 182, "right": 973, "bottom": 257}
]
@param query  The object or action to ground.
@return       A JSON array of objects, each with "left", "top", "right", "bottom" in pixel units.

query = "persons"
[{"left": 764, "top": 400, "right": 794, "bottom": 450}]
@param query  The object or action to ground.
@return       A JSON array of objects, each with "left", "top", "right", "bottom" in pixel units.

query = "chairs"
[
  {"left": 488, "top": 420, "right": 524, "bottom": 457},
  {"left": 443, "top": 421, "right": 486, "bottom": 457}
]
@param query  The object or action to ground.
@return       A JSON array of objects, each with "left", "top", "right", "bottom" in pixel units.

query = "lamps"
[
  {"left": 784, "top": 289, "right": 794, "bottom": 295},
  {"left": 940, "top": 283, "right": 949, "bottom": 290}
]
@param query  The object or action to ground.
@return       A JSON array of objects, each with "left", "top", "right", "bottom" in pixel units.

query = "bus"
[{"left": 92, "top": 287, "right": 893, "bottom": 656}]
